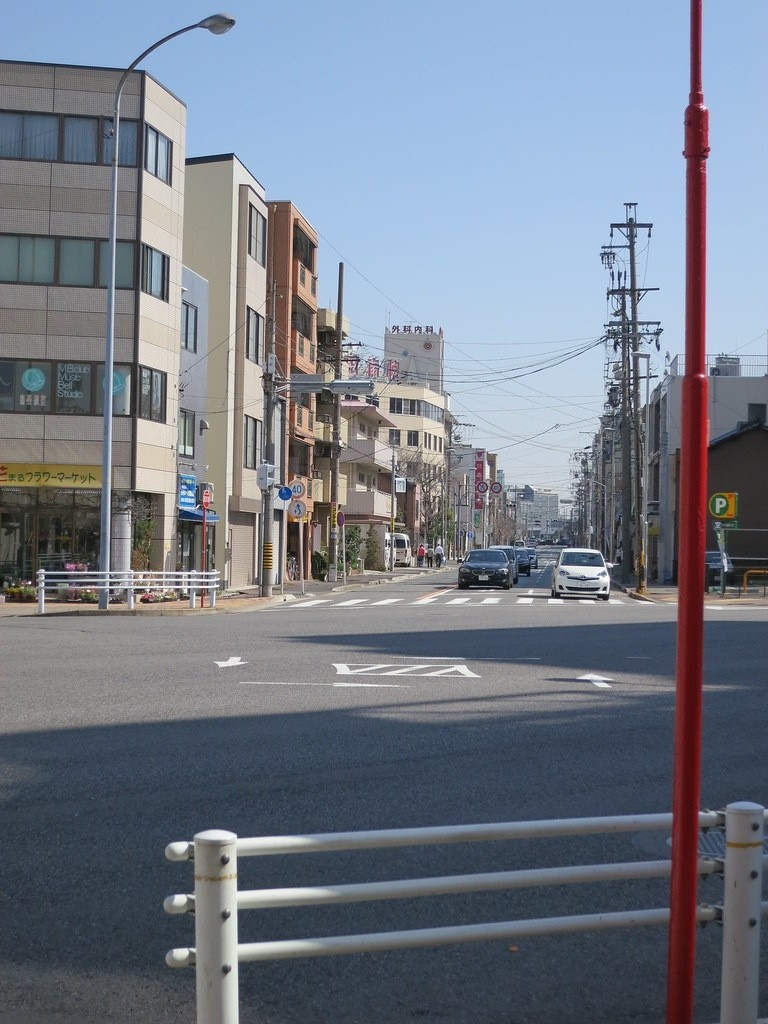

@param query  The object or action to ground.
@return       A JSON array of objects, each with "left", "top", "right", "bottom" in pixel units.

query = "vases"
[{"left": 142, "top": 599, "right": 159, "bottom": 603}]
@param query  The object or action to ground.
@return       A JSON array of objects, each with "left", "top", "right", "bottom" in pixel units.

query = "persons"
[
  {"left": 616, "top": 546, "right": 623, "bottom": 565},
  {"left": 472, "top": 553, "right": 482, "bottom": 561},
  {"left": 415, "top": 544, "right": 444, "bottom": 568},
  {"left": 563, "top": 555, "right": 575, "bottom": 565}
]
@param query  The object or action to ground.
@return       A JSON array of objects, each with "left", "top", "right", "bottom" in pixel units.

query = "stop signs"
[{"left": 202, "top": 489, "right": 211, "bottom": 509}]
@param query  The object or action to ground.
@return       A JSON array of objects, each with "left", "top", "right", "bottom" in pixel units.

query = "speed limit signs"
[
  {"left": 288, "top": 480, "right": 307, "bottom": 500},
  {"left": 491, "top": 481, "right": 501, "bottom": 493}
]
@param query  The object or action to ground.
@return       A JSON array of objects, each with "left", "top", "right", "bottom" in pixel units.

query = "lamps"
[{"left": 200, "top": 419, "right": 210, "bottom": 429}]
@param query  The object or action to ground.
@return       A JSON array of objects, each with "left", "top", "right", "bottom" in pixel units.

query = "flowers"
[{"left": 140, "top": 593, "right": 160, "bottom": 599}]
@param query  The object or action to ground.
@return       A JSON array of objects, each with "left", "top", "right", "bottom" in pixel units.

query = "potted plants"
[
  {"left": 312, "top": 524, "right": 379, "bottom": 581},
  {"left": 5, "top": 587, "right": 36, "bottom": 603},
  {"left": 80, "top": 593, "right": 97, "bottom": 602},
  {"left": 162, "top": 592, "right": 176, "bottom": 601}
]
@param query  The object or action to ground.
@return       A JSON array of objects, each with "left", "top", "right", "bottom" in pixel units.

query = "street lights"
[
  {"left": 629, "top": 350, "right": 652, "bottom": 591},
  {"left": 101, "top": 12, "right": 238, "bottom": 610},
  {"left": 603, "top": 426, "right": 631, "bottom": 583},
  {"left": 467, "top": 467, "right": 478, "bottom": 546},
  {"left": 580, "top": 476, "right": 607, "bottom": 561}
]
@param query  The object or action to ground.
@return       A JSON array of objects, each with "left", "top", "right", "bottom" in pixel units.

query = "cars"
[
  {"left": 551, "top": 547, "right": 614, "bottom": 600},
  {"left": 515, "top": 548, "right": 534, "bottom": 577},
  {"left": 457, "top": 549, "right": 514, "bottom": 589},
  {"left": 491, "top": 546, "right": 520, "bottom": 583},
  {"left": 525, "top": 547, "right": 540, "bottom": 569},
  {"left": 705, "top": 551, "right": 736, "bottom": 585}
]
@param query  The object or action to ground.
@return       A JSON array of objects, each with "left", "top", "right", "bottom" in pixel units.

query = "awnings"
[{"left": 178, "top": 507, "right": 220, "bottom": 522}]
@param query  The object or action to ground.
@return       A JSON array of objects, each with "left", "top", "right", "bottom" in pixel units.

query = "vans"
[
  {"left": 385, "top": 532, "right": 397, "bottom": 571},
  {"left": 513, "top": 540, "right": 525, "bottom": 549},
  {"left": 391, "top": 533, "right": 412, "bottom": 567}
]
